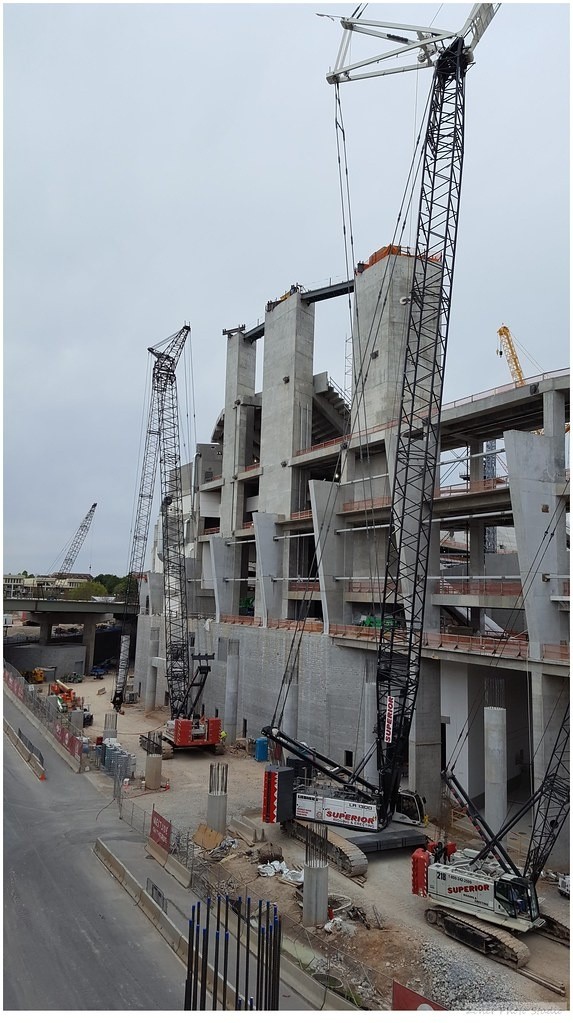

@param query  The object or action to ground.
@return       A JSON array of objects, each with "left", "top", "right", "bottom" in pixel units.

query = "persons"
[{"left": 510, "top": 888, "right": 527, "bottom": 914}]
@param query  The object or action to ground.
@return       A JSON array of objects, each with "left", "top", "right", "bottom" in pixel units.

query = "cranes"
[{"left": 497, "top": 323, "right": 570, "bottom": 438}]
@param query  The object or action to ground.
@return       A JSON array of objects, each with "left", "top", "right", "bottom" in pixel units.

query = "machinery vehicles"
[
  {"left": 109, "top": 317, "right": 226, "bottom": 760},
  {"left": 21, "top": 668, "right": 44, "bottom": 685},
  {"left": 20, "top": 503, "right": 97, "bottom": 626},
  {"left": 260, "top": 2, "right": 501, "bottom": 891},
  {"left": 49, "top": 680, "right": 83, "bottom": 712},
  {"left": 411, "top": 473, "right": 570, "bottom": 997}
]
